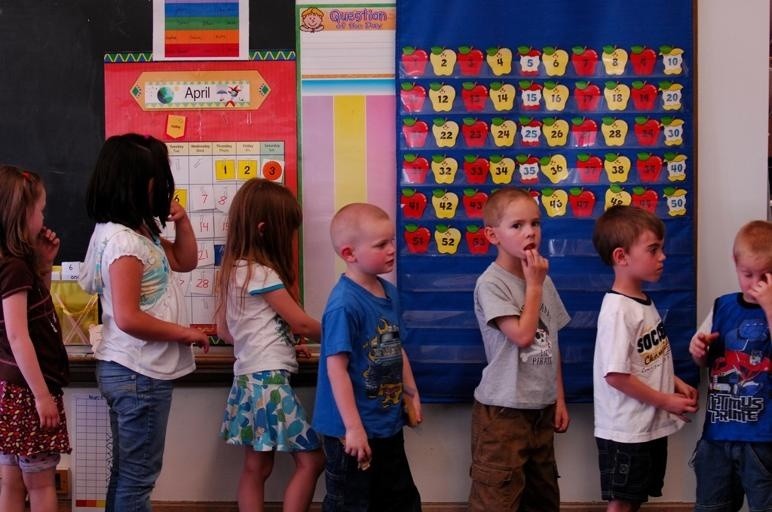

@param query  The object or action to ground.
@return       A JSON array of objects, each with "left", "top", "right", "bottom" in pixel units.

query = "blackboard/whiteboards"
[{"left": 0, "top": 0, "right": 770, "bottom": 365}]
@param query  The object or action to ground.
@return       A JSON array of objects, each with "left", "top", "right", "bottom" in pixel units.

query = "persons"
[
  {"left": 76, "top": 131, "right": 211, "bottom": 512},
  {"left": 0, "top": 161, "right": 73, "bottom": 512},
  {"left": 211, "top": 176, "right": 329, "bottom": 512},
  {"left": 466, "top": 187, "right": 572, "bottom": 512},
  {"left": 590, "top": 207, "right": 702, "bottom": 512},
  {"left": 309, "top": 201, "right": 424, "bottom": 512},
  {"left": 689, "top": 219, "right": 771, "bottom": 512}
]
